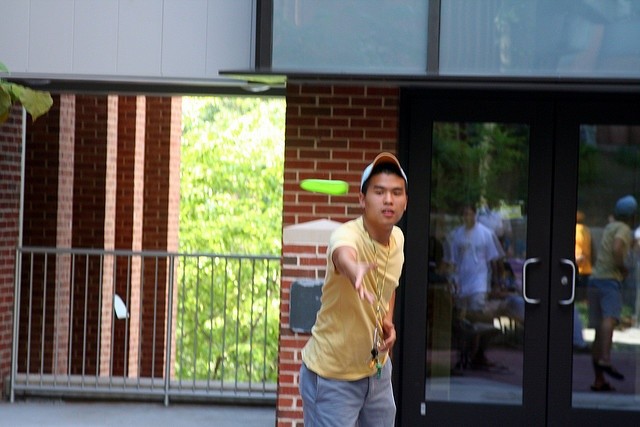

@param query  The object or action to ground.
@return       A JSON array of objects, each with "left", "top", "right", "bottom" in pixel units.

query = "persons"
[
  {"left": 585, "top": 196, "right": 637, "bottom": 392},
  {"left": 442, "top": 203, "right": 508, "bottom": 371},
  {"left": 477, "top": 196, "right": 509, "bottom": 298},
  {"left": 298, "top": 151, "right": 410, "bottom": 426}
]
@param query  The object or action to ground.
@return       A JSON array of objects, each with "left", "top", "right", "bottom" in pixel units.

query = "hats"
[
  {"left": 614, "top": 195, "right": 636, "bottom": 216},
  {"left": 361, "top": 152, "right": 408, "bottom": 188}
]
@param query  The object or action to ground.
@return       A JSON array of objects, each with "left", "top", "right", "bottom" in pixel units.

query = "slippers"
[
  {"left": 597, "top": 364, "right": 624, "bottom": 380},
  {"left": 589, "top": 381, "right": 616, "bottom": 392}
]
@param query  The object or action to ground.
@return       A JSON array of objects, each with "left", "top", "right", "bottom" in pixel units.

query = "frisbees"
[{"left": 300, "top": 178, "right": 348, "bottom": 196}]
